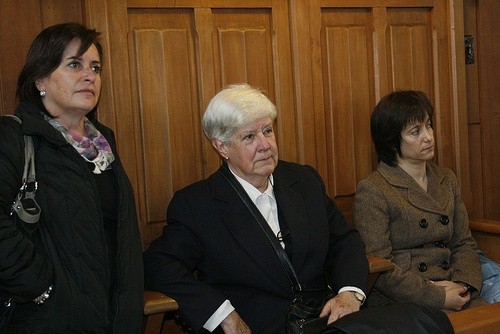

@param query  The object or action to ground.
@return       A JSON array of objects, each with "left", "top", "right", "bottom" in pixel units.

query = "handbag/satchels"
[
  {"left": 288, "top": 286, "right": 369, "bottom": 334},
  {"left": 0, "top": 114, "right": 42, "bottom": 334},
  {"left": 476, "top": 253, "right": 500, "bottom": 304}
]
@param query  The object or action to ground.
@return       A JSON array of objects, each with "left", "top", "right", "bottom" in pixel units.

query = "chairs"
[{"left": 145, "top": 254, "right": 394, "bottom": 334}]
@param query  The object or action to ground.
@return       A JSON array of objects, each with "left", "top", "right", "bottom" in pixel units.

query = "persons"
[
  {"left": 350, "top": 89, "right": 500, "bottom": 312},
  {"left": 142, "top": 84, "right": 454, "bottom": 334},
  {"left": 0, "top": 20, "right": 145, "bottom": 334}
]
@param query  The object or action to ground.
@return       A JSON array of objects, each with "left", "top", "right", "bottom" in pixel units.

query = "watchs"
[{"left": 352, "top": 289, "right": 364, "bottom": 302}]
